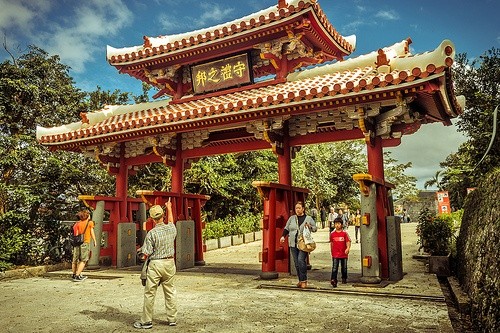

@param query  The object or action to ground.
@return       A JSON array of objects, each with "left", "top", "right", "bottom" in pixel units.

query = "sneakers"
[
  {"left": 161, "top": 320, "right": 177, "bottom": 325},
  {"left": 134, "top": 321, "right": 153, "bottom": 329}
]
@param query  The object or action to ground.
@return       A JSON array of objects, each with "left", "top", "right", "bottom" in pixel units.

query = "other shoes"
[
  {"left": 297, "top": 280, "right": 307, "bottom": 289},
  {"left": 72, "top": 274, "right": 88, "bottom": 281},
  {"left": 342, "top": 278, "right": 346, "bottom": 284},
  {"left": 331, "top": 279, "right": 337, "bottom": 287}
]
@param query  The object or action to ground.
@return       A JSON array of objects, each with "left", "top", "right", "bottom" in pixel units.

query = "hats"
[{"left": 149, "top": 205, "right": 163, "bottom": 218}]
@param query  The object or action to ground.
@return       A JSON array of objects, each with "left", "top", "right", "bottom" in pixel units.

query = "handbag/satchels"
[
  {"left": 70, "top": 234, "right": 84, "bottom": 247},
  {"left": 297, "top": 233, "right": 316, "bottom": 252}
]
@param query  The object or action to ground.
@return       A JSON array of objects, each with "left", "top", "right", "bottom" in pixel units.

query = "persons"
[
  {"left": 280, "top": 201, "right": 317, "bottom": 289},
  {"left": 354, "top": 208, "right": 362, "bottom": 243},
  {"left": 70, "top": 209, "right": 97, "bottom": 282},
  {"left": 403, "top": 208, "right": 407, "bottom": 223},
  {"left": 311, "top": 206, "right": 355, "bottom": 242},
  {"left": 330, "top": 218, "right": 351, "bottom": 288},
  {"left": 134, "top": 197, "right": 178, "bottom": 329}
]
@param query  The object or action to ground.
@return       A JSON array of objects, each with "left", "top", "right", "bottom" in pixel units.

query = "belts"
[{"left": 150, "top": 256, "right": 174, "bottom": 260}]
